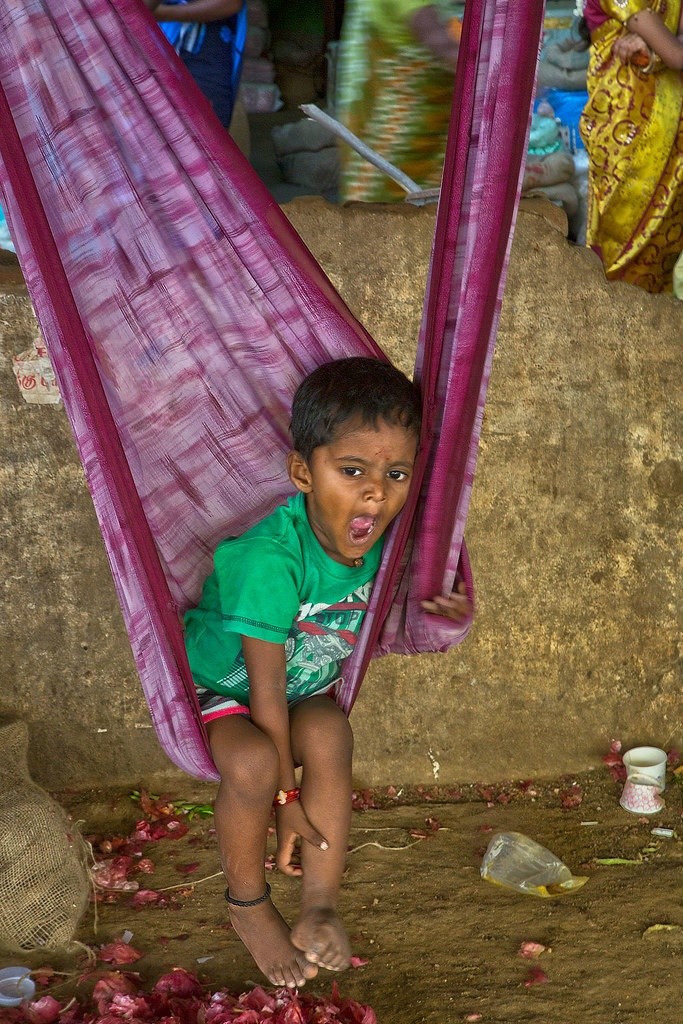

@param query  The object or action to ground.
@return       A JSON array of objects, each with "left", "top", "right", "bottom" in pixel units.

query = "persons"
[
  {"left": 177, "top": 358, "right": 473, "bottom": 988},
  {"left": 139, "top": 0, "right": 683, "bottom": 296}
]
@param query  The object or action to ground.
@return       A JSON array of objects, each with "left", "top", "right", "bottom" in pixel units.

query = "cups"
[
  {"left": 620, "top": 775, "right": 665, "bottom": 814},
  {"left": 0, "top": 977, "right": 35, "bottom": 1007},
  {"left": 621, "top": 746, "right": 668, "bottom": 792}
]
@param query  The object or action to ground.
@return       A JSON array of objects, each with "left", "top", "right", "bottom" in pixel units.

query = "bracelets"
[{"left": 273, "top": 784, "right": 300, "bottom": 810}]
